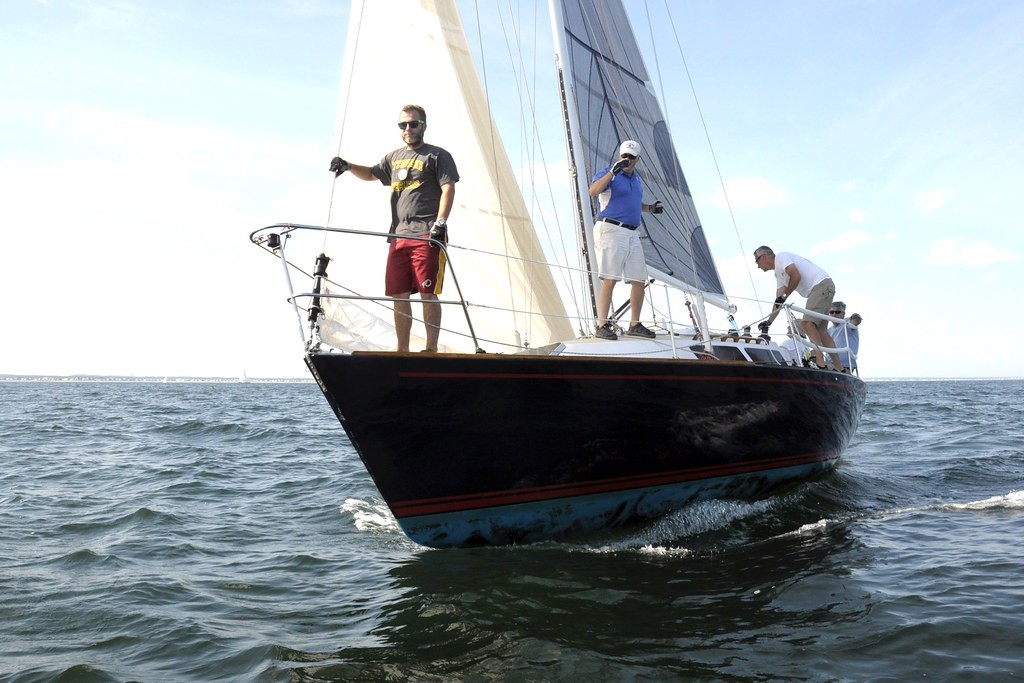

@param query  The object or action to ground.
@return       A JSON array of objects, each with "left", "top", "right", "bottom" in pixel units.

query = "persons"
[
  {"left": 590, "top": 140, "right": 663, "bottom": 340},
  {"left": 780, "top": 318, "right": 810, "bottom": 367},
  {"left": 825, "top": 302, "right": 862, "bottom": 374},
  {"left": 328, "top": 104, "right": 459, "bottom": 352},
  {"left": 754, "top": 246, "right": 846, "bottom": 374}
]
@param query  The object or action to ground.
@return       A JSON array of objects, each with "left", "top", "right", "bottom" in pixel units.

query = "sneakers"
[
  {"left": 596, "top": 325, "right": 617, "bottom": 340},
  {"left": 628, "top": 322, "right": 656, "bottom": 338}
]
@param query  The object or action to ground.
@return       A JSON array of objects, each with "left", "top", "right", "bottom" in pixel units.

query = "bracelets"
[{"left": 783, "top": 293, "right": 788, "bottom": 298}]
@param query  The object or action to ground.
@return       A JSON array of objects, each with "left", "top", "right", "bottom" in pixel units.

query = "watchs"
[{"left": 436, "top": 218, "right": 445, "bottom": 225}]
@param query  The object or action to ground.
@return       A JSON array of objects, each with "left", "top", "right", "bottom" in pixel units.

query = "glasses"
[
  {"left": 398, "top": 120, "right": 425, "bottom": 129},
  {"left": 622, "top": 154, "right": 636, "bottom": 159},
  {"left": 830, "top": 310, "right": 842, "bottom": 314},
  {"left": 756, "top": 253, "right": 765, "bottom": 263}
]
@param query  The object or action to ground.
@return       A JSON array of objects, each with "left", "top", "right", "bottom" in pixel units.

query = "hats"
[
  {"left": 788, "top": 319, "right": 806, "bottom": 339},
  {"left": 620, "top": 140, "right": 641, "bottom": 157}
]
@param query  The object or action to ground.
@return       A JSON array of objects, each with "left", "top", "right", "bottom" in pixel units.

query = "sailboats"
[{"left": 249, "top": 1, "right": 868, "bottom": 549}]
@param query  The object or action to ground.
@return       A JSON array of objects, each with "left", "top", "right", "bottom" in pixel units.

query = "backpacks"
[{"left": 610, "top": 158, "right": 630, "bottom": 176}]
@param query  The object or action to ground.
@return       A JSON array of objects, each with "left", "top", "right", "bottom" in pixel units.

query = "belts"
[{"left": 597, "top": 217, "right": 637, "bottom": 230}]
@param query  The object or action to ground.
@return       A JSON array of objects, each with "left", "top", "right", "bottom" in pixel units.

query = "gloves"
[
  {"left": 329, "top": 157, "right": 352, "bottom": 179},
  {"left": 758, "top": 319, "right": 771, "bottom": 329},
  {"left": 429, "top": 222, "right": 447, "bottom": 248},
  {"left": 649, "top": 201, "right": 663, "bottom": 214},
  {"left": 775, "top": 295, "right": 786, "bottom": 308}
]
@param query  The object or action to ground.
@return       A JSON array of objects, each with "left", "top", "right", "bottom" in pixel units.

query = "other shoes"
[
  {"left": 832, "top": 367, "right": 846, "bottom": 374},
  {"left": 817, "top": 364, "right": 828, "bottom": 370}
]
